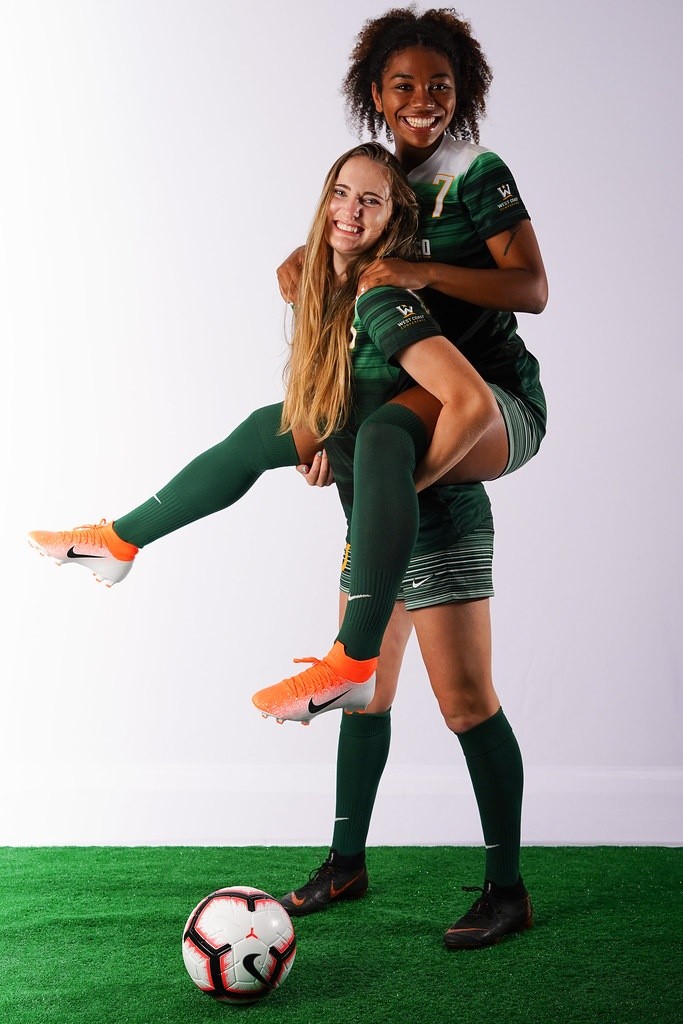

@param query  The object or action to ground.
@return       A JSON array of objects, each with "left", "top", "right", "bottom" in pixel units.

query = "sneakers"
[
  {"left": 28, "top": 517, "right": 138, "bottom": 588},
  {"left": 442, "top": 875, "right": 533, "bottom": 949},
  {"left": 252, "top": 638, "right": 376, "bottom": 725},
  {"left": 281, "top": 848, "right": 369, "bottom": 915}
]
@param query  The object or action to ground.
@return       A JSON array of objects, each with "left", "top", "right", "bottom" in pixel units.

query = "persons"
[
  {"left": 24, "top": 3, "right": 552, "bottom": 726},
  {"left": 277, "top": 143, "right": 536, "bottom": 947}
]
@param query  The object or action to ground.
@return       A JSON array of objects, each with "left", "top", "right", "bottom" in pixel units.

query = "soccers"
[{"left": 181, "top": 886, "right": 297, "bottom": 1006}]
